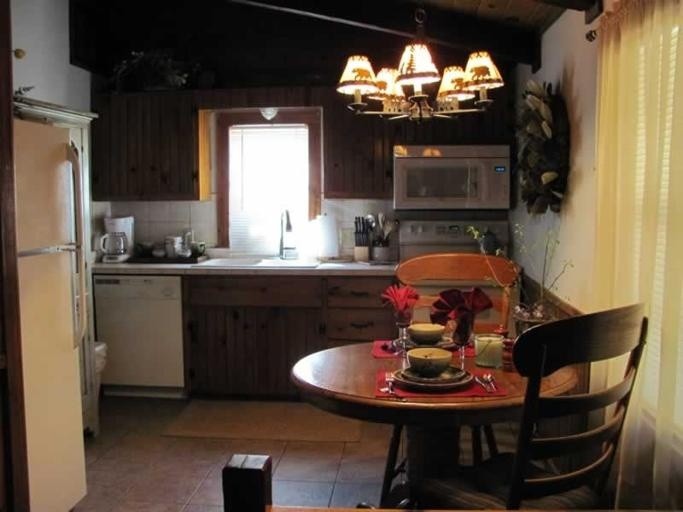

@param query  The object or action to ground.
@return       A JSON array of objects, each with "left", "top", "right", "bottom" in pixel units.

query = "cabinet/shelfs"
[
  {"left": 89, "top": 93, "right": 213, "bottom": 203},
  {"left": 182, "top": 272, "right": 324, "bottom": 403},
  {"left": 320, "top": 86, "right": 393, "bottom": 200},
  {"left": 324, "top": 276, "right": 398, "bottom": 349},
  {"left": 12, "top": 94, "right": 103, "bottom": 440},
  {"left": 391, "top": 81, "right": 516, "bottom": 146}
]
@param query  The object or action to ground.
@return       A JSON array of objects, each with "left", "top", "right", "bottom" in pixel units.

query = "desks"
[{"left": 290, "top": 340, "right": 579, "bottom": 506}]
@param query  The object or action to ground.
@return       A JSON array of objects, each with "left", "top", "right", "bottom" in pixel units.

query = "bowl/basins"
[{"left": 405, "top": 322, "right": 453, "bottom": 377}]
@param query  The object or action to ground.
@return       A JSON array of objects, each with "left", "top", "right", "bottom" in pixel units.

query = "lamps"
[{"left": 335, "top": 8, "right": 505, "bottom": 121}]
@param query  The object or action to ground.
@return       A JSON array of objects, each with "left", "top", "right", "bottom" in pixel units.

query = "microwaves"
[{"left": 392, "top": 145, "right": 510, "bottom": 211}]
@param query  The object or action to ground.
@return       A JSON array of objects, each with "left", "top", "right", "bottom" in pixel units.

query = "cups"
[
  {"left": 475, "top": 333, "right": 504, "bottom": 368},
  {"left": 138, "top": 231, "right": 206, "bottom": 258},
  {"left": 372, "top": 241, "right": 389, "bottom": 260}
]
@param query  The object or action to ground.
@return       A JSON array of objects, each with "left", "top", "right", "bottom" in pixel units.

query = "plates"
[{"left": 392, "top": 336, "right": 475, "bottom": 390}]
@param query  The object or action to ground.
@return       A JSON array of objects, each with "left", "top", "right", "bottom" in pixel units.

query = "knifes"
[{"left": 353, "top": 216, "right": 368, "bottom": 246}]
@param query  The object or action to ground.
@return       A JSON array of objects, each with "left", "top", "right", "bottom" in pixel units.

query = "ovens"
[{"left": 399, "top": 276, "right": 521, "bottom": 339}]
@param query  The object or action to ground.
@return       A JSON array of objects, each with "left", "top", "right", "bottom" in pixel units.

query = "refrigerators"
[{"left": 13, "top": 118, "right": 87, "bottom": 512}]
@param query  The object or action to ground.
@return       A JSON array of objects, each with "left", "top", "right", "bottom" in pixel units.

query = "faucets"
[{"left": 279, "top": 209, "right": 293, "bottom": 260}]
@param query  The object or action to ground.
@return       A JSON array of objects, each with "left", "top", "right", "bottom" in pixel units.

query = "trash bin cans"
[{"left": 94, "top": 341, "right": 107, "bottom": 406}]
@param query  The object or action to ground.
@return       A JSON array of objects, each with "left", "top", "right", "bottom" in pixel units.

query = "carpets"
[{"left": 161, "top": 400, "right": 363, "bottom": 442}]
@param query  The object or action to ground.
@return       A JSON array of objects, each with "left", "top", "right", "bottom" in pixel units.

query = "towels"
[
  {"left": 380, "top": 284, "right": 419, "bottom": 325},
  {"left": 428, "top": 286, "right": 493, "bottom": 345}
]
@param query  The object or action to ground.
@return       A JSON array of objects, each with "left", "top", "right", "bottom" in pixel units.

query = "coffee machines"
[{"left": 100, "top": 216, "right": 134, "bottom": 262}]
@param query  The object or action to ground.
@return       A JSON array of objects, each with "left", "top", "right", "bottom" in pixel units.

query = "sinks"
[
  {"left": 191, "top": 257, "right": 264, "bottom": 267},
  {"left": 256, "top": 258, "right": 323, "bottom": 268}
]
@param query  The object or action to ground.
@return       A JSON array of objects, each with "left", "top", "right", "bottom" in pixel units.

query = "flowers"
[
  {"left": 512, "top": 301, "right": 545, "bottom": 321},
  {"left": 512, "top": 73, "right": 570, "bottom": 217}
]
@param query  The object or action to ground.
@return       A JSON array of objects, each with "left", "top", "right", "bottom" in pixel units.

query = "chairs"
[
  {"left": 424, "top": 302, "right": 650, "bottom": 511},
  {"left": 377, "top": 253, "right": 521, "bottom": 507}
]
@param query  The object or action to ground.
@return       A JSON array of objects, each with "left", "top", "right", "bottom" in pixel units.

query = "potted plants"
[{"left": 466, "top": 221, "right": 574, "bottom": 338}]
